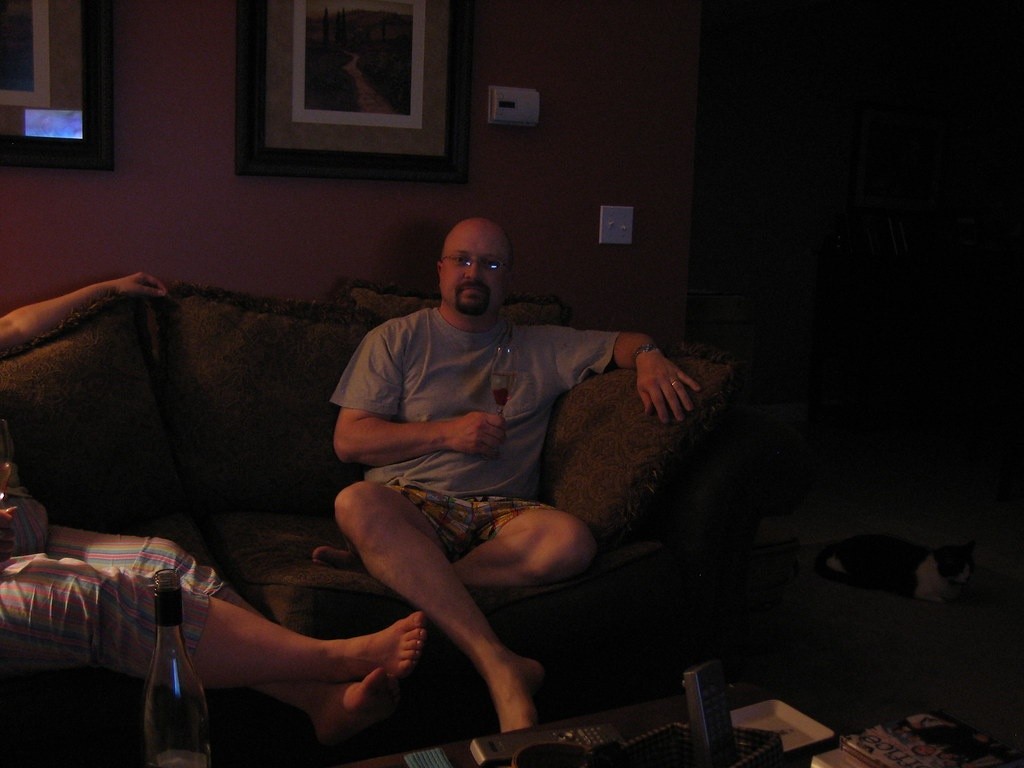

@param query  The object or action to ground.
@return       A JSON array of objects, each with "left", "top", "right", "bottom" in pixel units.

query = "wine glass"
[
  {"left": 0, "top": 419, "right": 14, "bottom": 513},
  {"left": 482, "top": 344, "right": 521, "bottom": 462}
]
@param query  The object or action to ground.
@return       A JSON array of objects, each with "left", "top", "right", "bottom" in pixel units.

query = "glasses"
[{"left": 441, "top": 255, "right": 510, "bottom": 273}]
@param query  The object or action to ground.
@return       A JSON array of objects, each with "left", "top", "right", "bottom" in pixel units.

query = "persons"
[
  {"left": 0, "top": 271, "right": 426, "bottom": 747},
  {"left": 316, "top": 218, "right": 701, "bottom": 733}
]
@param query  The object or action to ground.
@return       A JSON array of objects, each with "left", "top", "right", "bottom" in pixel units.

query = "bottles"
[{"left": 143, "top": 569, "right": 211, "bottom": 768}]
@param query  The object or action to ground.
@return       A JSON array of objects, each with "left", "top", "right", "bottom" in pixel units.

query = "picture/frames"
[
  {"left": 235, "top": 0, "right": 473, "bottom": 184},
  {"left": 0, "top": 0, "right": 116, "bottom": 172}
]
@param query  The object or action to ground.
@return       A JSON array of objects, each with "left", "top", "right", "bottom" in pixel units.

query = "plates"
[{"left": 730, "top": 698, "right": 835, "bottom": 753}]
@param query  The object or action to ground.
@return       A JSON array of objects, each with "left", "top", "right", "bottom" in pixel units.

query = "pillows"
[{"left": 536, "top": 338, "right": 740, "bottom": 549}]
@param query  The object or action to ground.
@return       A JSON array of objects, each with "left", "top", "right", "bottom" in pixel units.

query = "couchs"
[{"left": 0, "top": 281, "right": 821, "bottom": 767}]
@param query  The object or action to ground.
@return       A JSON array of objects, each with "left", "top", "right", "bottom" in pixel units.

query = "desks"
[{"left": 330, "top": 681, "right": 819, "bottom": 767}]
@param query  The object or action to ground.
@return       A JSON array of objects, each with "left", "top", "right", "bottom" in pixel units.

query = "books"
[{"left": 810, "top": 709, "right": 1024, "bottom": 768}]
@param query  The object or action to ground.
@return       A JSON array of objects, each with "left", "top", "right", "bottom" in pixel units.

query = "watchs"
[{"left": 631, "top": 343, "right": 657, "bottom": 370}]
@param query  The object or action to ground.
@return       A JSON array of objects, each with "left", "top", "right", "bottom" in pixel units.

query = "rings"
[{"left": 671, "top": 378, "right": 679, "bottom": 386}]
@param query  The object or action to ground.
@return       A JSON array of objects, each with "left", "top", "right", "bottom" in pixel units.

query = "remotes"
[
  {"left": 469, "top": 723, "right": 627, "bottom": 767},
  {"left": 684, "top": 659, "right": 738, "bottom": 768}
]
